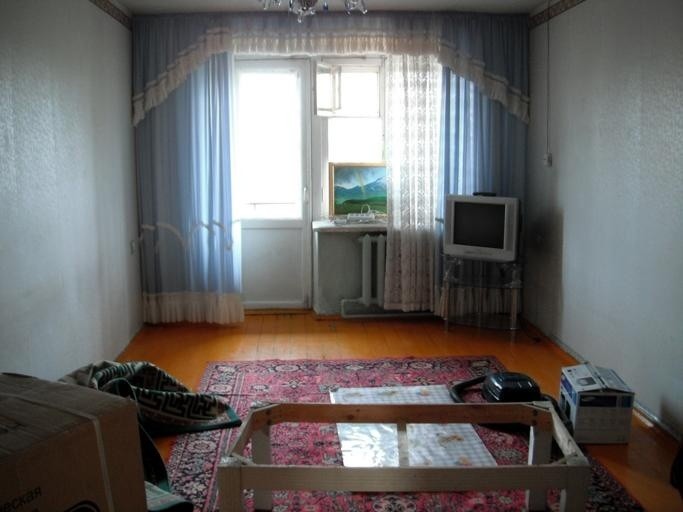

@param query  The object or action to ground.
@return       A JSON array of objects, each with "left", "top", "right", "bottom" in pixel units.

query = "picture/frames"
[{"left": 329, "top": 161, "right": 387, "bottom": 221}]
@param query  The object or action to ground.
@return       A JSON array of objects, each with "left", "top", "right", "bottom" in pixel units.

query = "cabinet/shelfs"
[{"left": 440, "top": 253, "right": 522, "bottom": 342}]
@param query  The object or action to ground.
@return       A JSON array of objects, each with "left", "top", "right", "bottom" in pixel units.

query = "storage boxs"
[
  {"left": 0, "top": 369, "right": 148, "bottom": 510},
  {"left": 558, "top": 363, "right": 634, "bottom": 445}
]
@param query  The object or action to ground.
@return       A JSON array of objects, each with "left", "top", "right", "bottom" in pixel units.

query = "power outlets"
[{"left": 542, "top": 153, "right": 551, "bottom": 167}]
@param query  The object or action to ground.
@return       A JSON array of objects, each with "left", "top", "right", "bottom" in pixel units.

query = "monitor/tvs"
[{"left": 443, "top": 194, "right": 523, "bottom": 263}]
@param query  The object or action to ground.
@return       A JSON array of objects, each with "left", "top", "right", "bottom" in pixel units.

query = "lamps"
[{"left": 257, "top": 0, "right": 369, "bottom": 24}]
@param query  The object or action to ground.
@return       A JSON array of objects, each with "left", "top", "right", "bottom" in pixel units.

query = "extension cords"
[{"left": 347, "top": 213, "right": 375, "bottom": 222}]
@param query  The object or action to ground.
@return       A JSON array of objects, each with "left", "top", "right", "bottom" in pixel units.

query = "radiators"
[{"left": 358, "top": 233, "right": 386, "bottom": 307}]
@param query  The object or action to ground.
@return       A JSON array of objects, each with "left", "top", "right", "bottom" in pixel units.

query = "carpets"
[{"left": 159, "top": 358, "right": 648, "bottom": 511}]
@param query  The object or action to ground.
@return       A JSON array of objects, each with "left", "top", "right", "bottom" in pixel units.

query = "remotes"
[{"left": 472, "top": 191, "right": 497, "bottom": 196}]
